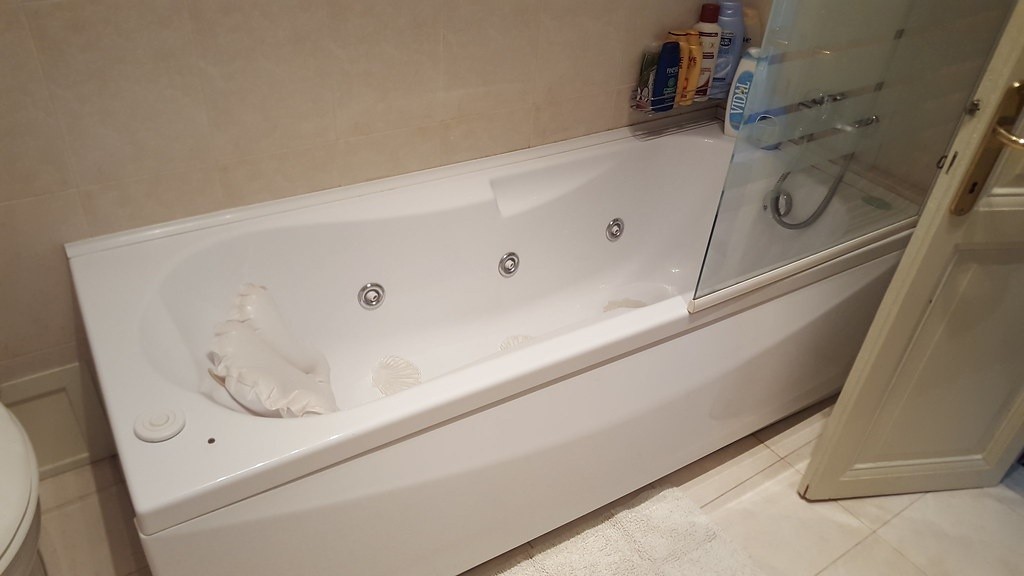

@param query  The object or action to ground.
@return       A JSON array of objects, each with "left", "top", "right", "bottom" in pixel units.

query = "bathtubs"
[{"left": 61, "top": 111, "right": 927, "bottom": 576}]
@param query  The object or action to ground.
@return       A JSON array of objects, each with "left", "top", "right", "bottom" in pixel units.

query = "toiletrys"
[{"left": 631, "top": 0, "right": 790, "bottom": 151}]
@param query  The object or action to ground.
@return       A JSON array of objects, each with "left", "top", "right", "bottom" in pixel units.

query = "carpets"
[{"left": 488, "top": 478, "right": 767, "bottom": 576}]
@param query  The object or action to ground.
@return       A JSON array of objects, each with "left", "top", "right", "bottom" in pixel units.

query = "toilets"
[{"left": 0, "top": 396, "right": 50, "bottom": 576}]
